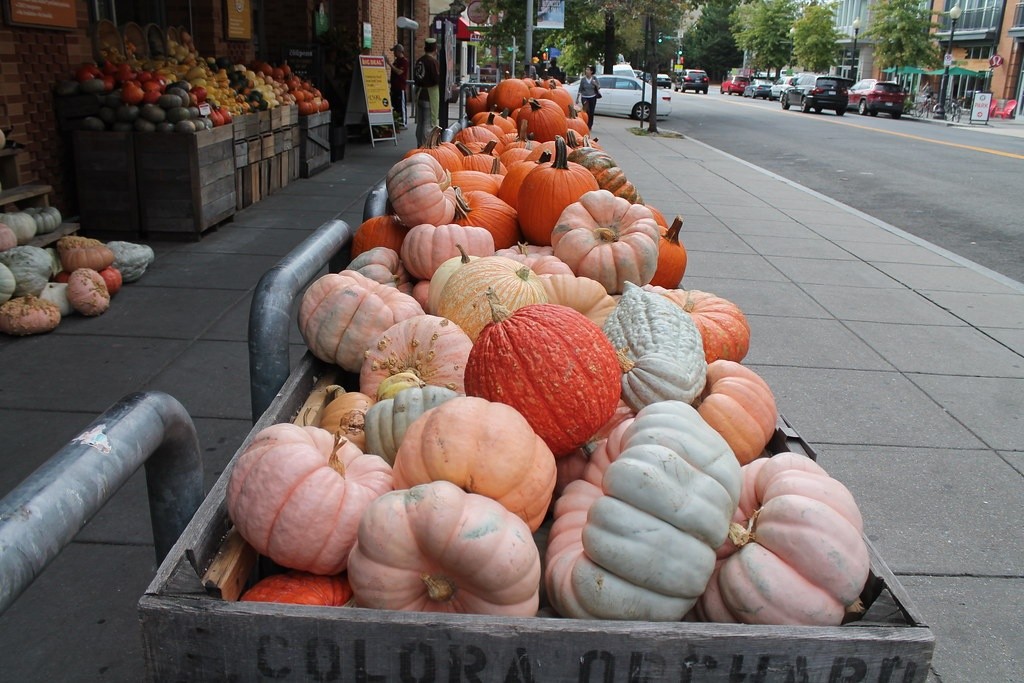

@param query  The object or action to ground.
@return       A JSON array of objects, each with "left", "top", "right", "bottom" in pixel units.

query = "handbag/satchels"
[{"left": 593, "top": 76, "right": 602, "bottom": 98}]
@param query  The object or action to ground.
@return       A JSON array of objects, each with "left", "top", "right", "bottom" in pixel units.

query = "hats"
[
  {"left": 425, "top": 38, "right": 436, "bottom": 46},
  {"left": 390, "top": 44, "right": 404, "bottom": 52}
]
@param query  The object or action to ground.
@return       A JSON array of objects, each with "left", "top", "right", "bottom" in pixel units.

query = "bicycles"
[
  {"left": 944, "top": 98, "right": 963, "bottom": 122},
  {"left": 914, "top": 95, "right": 935, "bottom": 117}
]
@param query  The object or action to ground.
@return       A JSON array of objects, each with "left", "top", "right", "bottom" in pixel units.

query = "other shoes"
[{"left": 398, "top": 125, "right": 408, "bottom": 130}]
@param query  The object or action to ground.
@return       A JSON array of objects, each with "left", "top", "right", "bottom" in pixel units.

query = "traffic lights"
[
  {"left": 678, "top": 44, "right": 683, "bottom": 56},
  {"left": 657, "top": 31, "right": 663, "bottom": 44}
]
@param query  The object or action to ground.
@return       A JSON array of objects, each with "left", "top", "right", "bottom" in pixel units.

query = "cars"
[
  {"left": 613, "top": 64, "right": 672, "bottom": 89},
  {"left": 561, "top": 75, "right": 671, "bottom": 121},
  {"left": 743, "top": 79, "right": 773, "bottom": 100},
  {"left": 720, "top": 76, "right": 750, "bottom": 96},
  {"left": 768, "top": 73, "right": 848, "bottom": 116},
  {"left": 674, "top": 69, "right": 709, "bottom": 94}
]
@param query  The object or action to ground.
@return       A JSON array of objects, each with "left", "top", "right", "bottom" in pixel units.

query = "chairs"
[{"left": 990, "top": 99, "right": 1017, "bottom": 118}]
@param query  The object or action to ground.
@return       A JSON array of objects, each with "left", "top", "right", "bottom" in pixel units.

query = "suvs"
[{"left": 847, "top": 79, "right": 905, "bottom": 119}]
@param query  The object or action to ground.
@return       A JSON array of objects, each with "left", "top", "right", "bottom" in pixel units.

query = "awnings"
[{"left": 457, "top": 16, "right": 484, "bottom": 41}]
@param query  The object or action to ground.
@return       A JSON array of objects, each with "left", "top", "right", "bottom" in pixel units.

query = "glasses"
[{"left": 587, "top": 65, "right": 593, "bottom": 68}]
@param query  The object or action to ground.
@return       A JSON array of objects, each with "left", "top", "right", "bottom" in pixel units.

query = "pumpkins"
[
  {"left": 0, "top": 207, "right": 154, "bottom": 335},
  {"left": 57, "top": 32, "right": 329, "bottom": 132},
  {"left": 226, "top": 78, "right": 869, "bottom": 627}
]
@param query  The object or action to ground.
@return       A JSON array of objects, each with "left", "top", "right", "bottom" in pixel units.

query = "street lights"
[
  {"left": 932, "top": 3, "right": 962, "bottom": 119},
  {"left": 790, "top": 27, "right": 795, "bottom": 69},
  {"left": 851, "top": 17, "right": 861, "bottom": 78}
]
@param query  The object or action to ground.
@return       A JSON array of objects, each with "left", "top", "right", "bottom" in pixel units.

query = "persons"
[
  {"left": 385, "top": 44, "right": 408, "bottom": 130},
  {"left": 414, "top": 37, "right": 439, "bottom": 149},
  {"left": 918, "top": 81, "right": 933, "bottom": 112},
  {"left": 576, "top": 64, "right": 600, "bottom": 129},
  {"left": 548, "top": 60, "right": 566, "bottom": 84}
]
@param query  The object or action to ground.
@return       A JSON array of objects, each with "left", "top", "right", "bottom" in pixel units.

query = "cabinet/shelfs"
[{"left": 1, "top": 148, "right": 81, "bottom": 250}]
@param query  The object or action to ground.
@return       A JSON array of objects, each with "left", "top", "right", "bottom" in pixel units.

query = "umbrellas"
[
  {"left": 921, "top": 66, "right": 981, "bottom": 98},
  {"left": 881, "top": 66, "right": 925, "bottom": 73}
]
